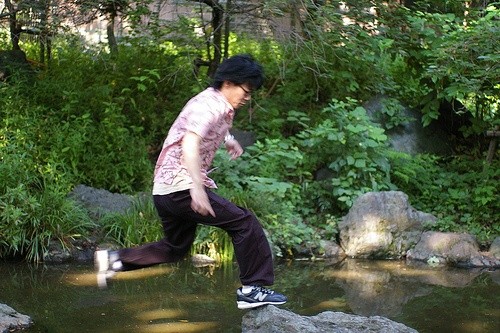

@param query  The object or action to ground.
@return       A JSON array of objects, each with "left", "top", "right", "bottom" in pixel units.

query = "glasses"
[{"left": 240, "top": 85, "right": 250, "bottom": 94}]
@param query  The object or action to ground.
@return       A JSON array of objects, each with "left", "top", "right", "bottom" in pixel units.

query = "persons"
[{"left": 93, "top": 54, "right": 287, "bottom": 309}]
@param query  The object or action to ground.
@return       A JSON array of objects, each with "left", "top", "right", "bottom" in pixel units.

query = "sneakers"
[
  {"left": 93, "top": 247, "right": 123, "bottom": 289},
  {"left": 236, "top": 288, "right": 286, "bottom": 310}
]
